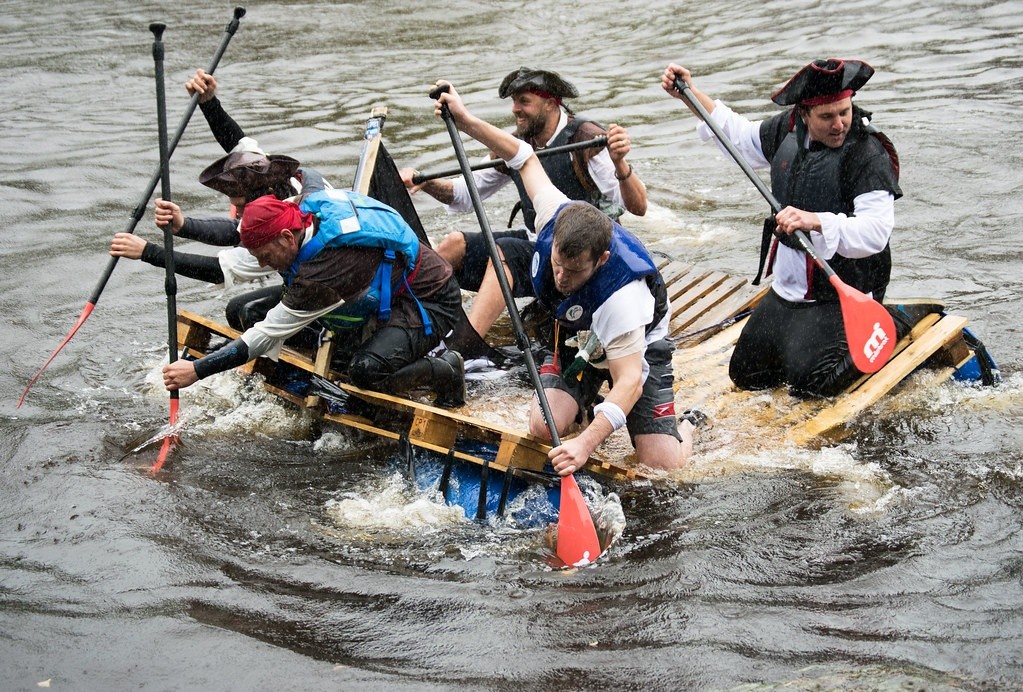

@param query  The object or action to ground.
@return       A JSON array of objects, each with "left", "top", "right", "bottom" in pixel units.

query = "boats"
[{"left": 167, "top": 255, "right": 1000, "bottom": 531}]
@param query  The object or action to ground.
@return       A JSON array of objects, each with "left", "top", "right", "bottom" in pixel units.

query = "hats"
[
  {"left": 198, "top": 151, "right": 300, "bottom": 198},
  {"left": 771, "top": 59, "right": 875, "bottom": 106},
  {"left": 498, "top": 66, "right": 580, "bottom": 99}
]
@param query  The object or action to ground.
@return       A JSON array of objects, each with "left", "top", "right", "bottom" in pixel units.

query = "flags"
[{"left": 332, "top": 139, "right": 525, "bottom": 369}]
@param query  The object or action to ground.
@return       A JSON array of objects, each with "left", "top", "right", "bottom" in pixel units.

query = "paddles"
[
  {"left": 425, "top": 87, "right": 603, "bottom": 566},
  {"left": 678, "top": 77, "right": 897, "bottom": 373},
  {"left": 145, "top": 25, "right": 182, "bottom": 475},
  {"left": 411, "top": 132, "right": 606, "bottom": 185},
  {"left": 17, "top": 6, "right": 249, "bottom": 409}
]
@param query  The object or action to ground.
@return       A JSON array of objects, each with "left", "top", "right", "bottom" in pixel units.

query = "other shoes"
[
  {"left": 880, "top": 298, "right": 946, "bottom": 330},
  {"left": 423, "top": 349, "right": 466, "bottom": 410},
  {"left": 678, "top": 409, "right": 708, "bottom": 429}
]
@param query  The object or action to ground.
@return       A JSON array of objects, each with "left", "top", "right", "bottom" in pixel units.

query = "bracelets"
[
  {"left": 614, "top": 163, "right": 632, "bottom": 179},
  {"left": 505, "top": 139, "right": 533, "bottom": 170},
  {"left": 593, "top": 402, "right": 627, "bottom": 433}
]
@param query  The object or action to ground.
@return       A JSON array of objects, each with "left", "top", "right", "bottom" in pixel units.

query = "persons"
[
  {"left": 155, "top": 190, "right": 466, "bottom": 409},
  {"left": 435, "top": 80, "right": 708, "bottom": 477},
  {"left": 110, "top": 69, "right": 335, "bottom": 289},
  {"left": 400, "top": 66, "right": 647, "bottom": 340},
  {"left": 662, "top": 59, "right": 945, "bottom": 400}
]
